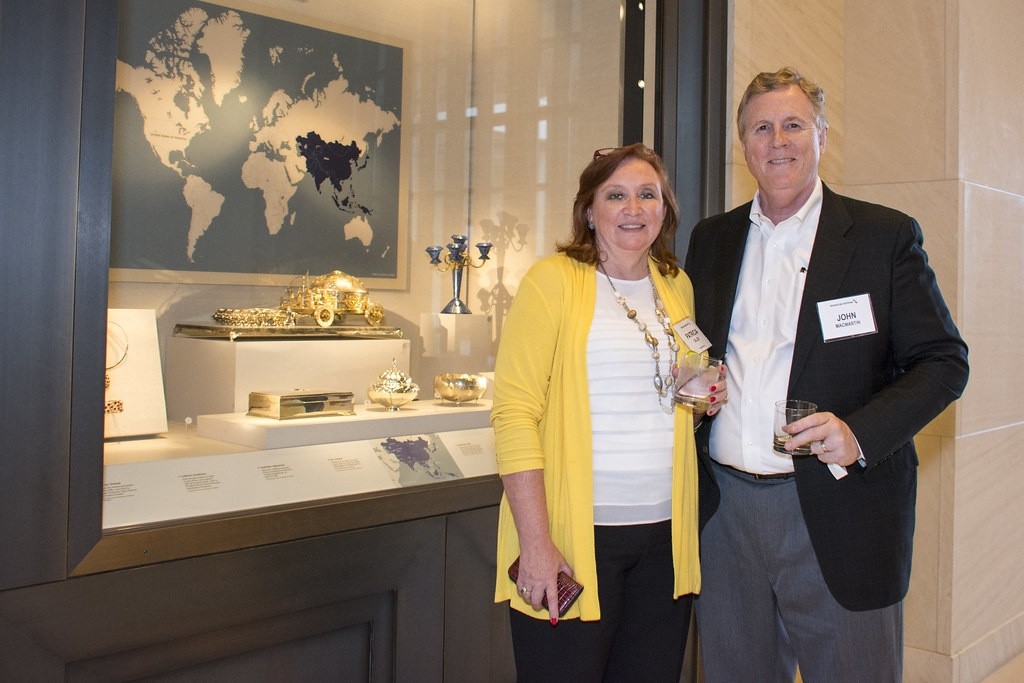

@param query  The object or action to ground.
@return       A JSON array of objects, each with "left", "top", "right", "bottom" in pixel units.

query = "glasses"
[{"left": 593, "top": 147, "right": 656, "bottom": 160}]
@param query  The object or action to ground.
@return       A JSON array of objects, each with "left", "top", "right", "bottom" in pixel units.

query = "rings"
[
  {"left": 820, "top": 441, "right": 831, "bottom": 454},
  {"left": 518, "top": 586, "right": 532, "bottom": 596},
  {"left": 724, "top": 395, "right": 730, "bottom": 401}
]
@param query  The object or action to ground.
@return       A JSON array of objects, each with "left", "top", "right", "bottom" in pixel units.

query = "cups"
[
  {"left": 772, "top": 401, "right": 817, "bottom": 455},
  {"left": 672, "top": 351, "right": 723, "bottom": 413}
]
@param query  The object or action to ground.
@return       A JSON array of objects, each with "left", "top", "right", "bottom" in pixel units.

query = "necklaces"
[{"left": 598, "top": 257, "right": 681, "bottom": 415}]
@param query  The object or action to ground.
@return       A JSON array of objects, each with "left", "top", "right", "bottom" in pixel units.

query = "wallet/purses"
[{"left": 507, "top": 556, "right": 584, "bottom": 618}]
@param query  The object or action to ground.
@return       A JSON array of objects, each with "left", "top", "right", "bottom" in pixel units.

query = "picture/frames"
[{"left": 108, "top": 0, "right": 413, "bottom": 289}]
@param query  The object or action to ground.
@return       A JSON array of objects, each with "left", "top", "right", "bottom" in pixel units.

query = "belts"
[{"left": 709, "top": 458, "right": 796, "bottom": 480}]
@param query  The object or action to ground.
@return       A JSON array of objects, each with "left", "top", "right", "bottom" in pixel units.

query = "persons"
[
  {"left": 488, "top": 142, "right": 729, "bottom": 683},
  {"left": 682, "top": 66, "right": 970, "bottom": 683}
]
